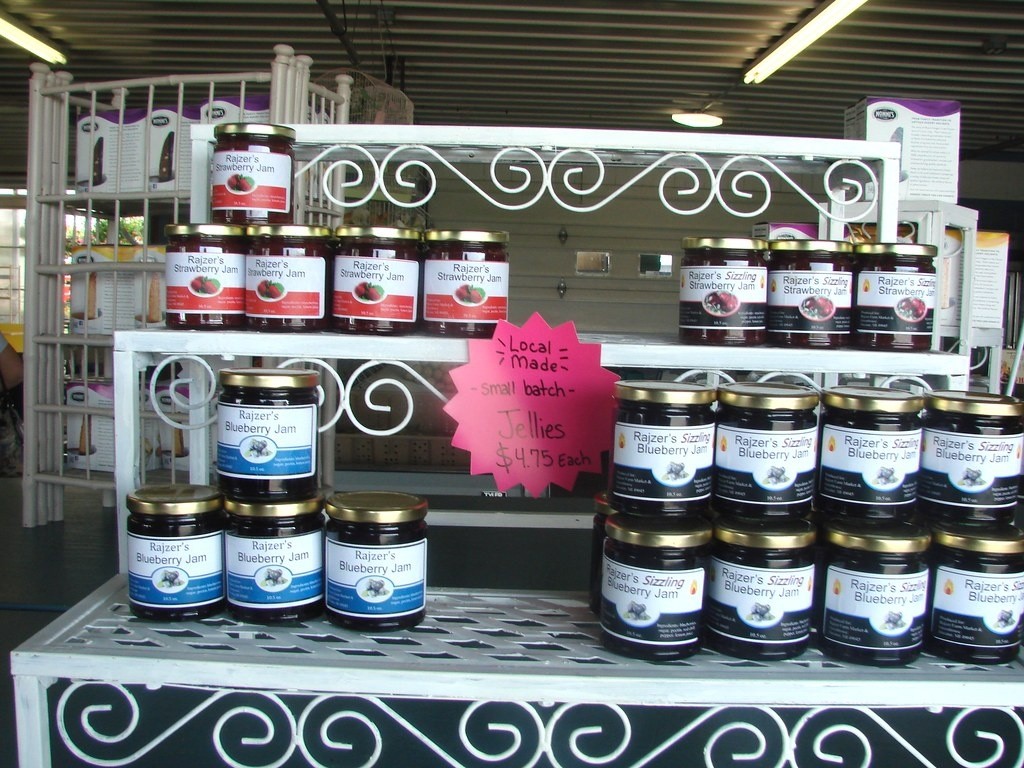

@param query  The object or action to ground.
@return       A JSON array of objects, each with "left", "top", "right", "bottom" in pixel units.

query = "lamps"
[
  {"left": 671, "top": 101, "right": 723, "bottom": 128},
  {"left": 743, "top": 0, "right": 869, "bottom": 83},
  {"left": 559, "top": 227, "right": 568, "bottom": 243},
  {"left": 558, "top": 277, "right": 568, "bottom": 299},
  {"left": 0, "top": 9, "right": 72, "bottom": 65}
]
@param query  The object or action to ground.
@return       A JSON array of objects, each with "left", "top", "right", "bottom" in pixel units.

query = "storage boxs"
[
  {"left": 843, "top": 95, "right": 959, "bottom": 204},
  {"left": 70, "top": 243, "right": 168, "bottom": 336},
  {"left": 156, "top": 379, "right": 218, "bottom": 474},
  {"left": 62, "top": 380, "right": 163, "bottom": 472},
  {"left": 751, "top": 220, "right": 851, "bottom": 243},
  {"left": 76, "top": 105, "right": 147, "bottom": 193},
  {"left": 850, "top": 224, "right": 1010, "bottom": 329},
  {"left": 144, "top": 102, "right": 200, "bottom": 192},
  {"left": 1, "top": 323, "right": 24, "bottom": 353},
  {"left": 197, "top": 93, "right": 275, "bottom": 124}
]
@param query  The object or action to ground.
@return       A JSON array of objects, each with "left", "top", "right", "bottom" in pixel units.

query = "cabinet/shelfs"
[
  {"left": 7, "top": 122, "right": 1021, "bottom": 768},
  {"left": 21, "top": 44, "right": 356, "bottom": 529}
]
